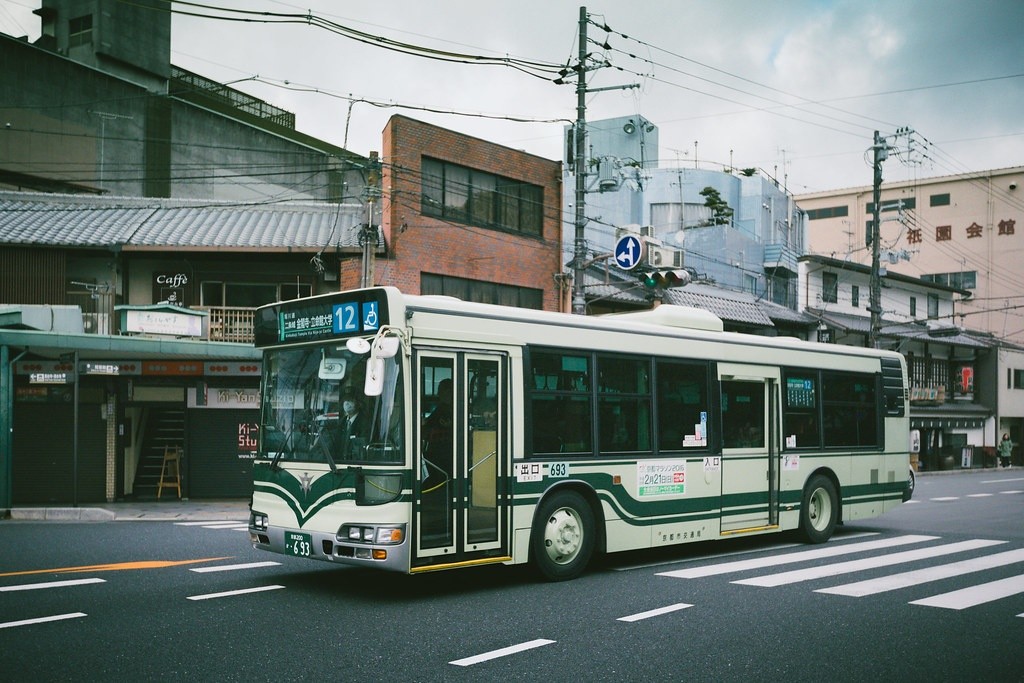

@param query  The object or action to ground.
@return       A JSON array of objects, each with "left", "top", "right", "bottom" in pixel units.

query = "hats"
[{"left": 341, "top": 386, "right": 364, "bottom": 403}]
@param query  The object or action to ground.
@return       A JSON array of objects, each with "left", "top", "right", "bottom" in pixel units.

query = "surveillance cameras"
[{"left": 1009, "top": 182, "right": 1016, "bottom": 189}]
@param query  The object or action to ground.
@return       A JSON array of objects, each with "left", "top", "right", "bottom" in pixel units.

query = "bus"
[{"left": 243, "top": 284, "right": 915, "bottom": 580}]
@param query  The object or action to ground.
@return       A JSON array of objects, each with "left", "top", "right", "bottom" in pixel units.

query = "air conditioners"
[
  {"left": 674, "top": 250, "right": 686, "bottom": 267},
  {"left": 614, "top": 224, "right": 640, "bottom": 245},
  {"left": 641, "top": 226, "right": 655, "bottom": 237},
  {"left": 82, "top": 312, "right": 110, "bottom": 335},
  {"left": 643, "top": 245, "right": 657, "bottom": 266}
]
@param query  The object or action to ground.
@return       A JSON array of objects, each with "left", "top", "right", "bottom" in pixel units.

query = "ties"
[{"left": 346, "top": 420, "right": 351, "bottom": 431}]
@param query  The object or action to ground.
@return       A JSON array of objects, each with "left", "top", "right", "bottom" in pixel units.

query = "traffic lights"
[{"left": 641, "top": 270, "right": 690, "bottom": 291}]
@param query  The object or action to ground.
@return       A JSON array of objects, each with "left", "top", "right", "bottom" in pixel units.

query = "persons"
[
  {"left": 999, "top": 433, "right": 1013, "bottom": 468},
  {"left": 330, "top": 387, "right": 377, "bottom": 460},
  {"left": 426, "top": 377, "right": 453, "bottom": 472}
]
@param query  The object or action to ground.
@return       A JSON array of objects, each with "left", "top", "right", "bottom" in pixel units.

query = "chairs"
[
  {"left": 532, "top": 401, "right": 562, "bottom": 453},
  {"left": 428, "top": 378, "right": 452, "bottom": 415},
  {"left": 560, "top": 399, "right": 590, "bottom": 452},
  {"left": 232, "top": 321, "right": 251, "bottom": 341}
]
